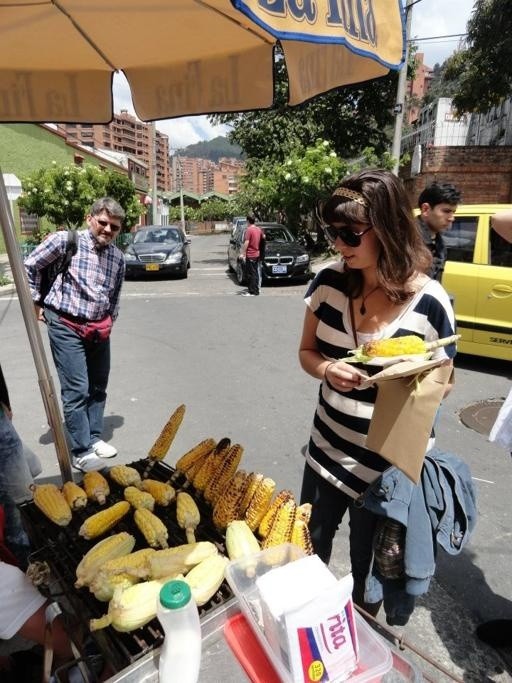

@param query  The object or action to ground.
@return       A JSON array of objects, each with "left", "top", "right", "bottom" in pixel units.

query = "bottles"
[{"left": 156, "top": 579, "right": 203, "bottom": 683}]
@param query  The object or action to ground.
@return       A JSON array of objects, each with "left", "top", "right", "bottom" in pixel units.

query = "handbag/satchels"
[
  {"left": 371, "top": 517, "right": 406, "bottom": 579},
  {"left": 40, "top": 230, "right": 78, "bottom": 296},
  {"left": 59, "top": 313, "right": 112, "bottom": 344}
]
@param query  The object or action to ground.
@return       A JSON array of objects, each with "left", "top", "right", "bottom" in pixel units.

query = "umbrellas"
[{"left": 0, "top": 0, "right": 408, "bottom": 490}]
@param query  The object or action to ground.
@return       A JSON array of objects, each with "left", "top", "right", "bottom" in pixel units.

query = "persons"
[
  {"left": 412, "top": 180, "right": 462, "bottom": 283},
  {"left": 239, "top": 214, "right": 266, "bottom": 294},
  {"left": 297, "top": 165, "right": 456, "bottom": 618},
  {"left": 0, "top": 365, "right": 42, "bottom": 557},
  {"left": 22, "top": 195, "right": 126, "bottom": 470},
  {"left": 0, "top": 561, "right": 75, "bottom": 682}
]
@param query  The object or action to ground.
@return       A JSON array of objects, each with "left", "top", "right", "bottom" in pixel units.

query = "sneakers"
[
  {"left": 72, "top": 451, "right": 106, "bottom": 473},
  {"left": 90, "top": 439, "right": 117, "bottom": 458}
]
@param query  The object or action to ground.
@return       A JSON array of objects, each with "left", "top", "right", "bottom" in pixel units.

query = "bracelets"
[
  {"left": 357, "top": 279, "right": 383, "bottom": 315},
  {"left": 325, "top": 362, "right": 332, "bottom": 379}
]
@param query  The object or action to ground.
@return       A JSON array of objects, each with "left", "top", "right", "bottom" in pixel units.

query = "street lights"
[
  {"left": 152, "top": 134, "right": 169, "bottom": 226},
  {"left": 177, "top": 153, "right": 186, "bottom": 234}
]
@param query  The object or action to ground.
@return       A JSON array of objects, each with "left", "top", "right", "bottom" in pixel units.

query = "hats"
[{"left": 247, "top": 211, "right": 255, "bottom": 220}]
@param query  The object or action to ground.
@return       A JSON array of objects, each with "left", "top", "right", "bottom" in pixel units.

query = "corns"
[
  {"left": 33, "top": 404, "right": 314, "bottom": 633},
  {"left": 362, "top": 335, "right": 428, "bottom": 358}
]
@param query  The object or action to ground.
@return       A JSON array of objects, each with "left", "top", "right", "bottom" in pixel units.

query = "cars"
[
  {"left": 120, "top": 224, "right": 193, "bottom": 281},
  {"left": 408, "top": 202, "right": 512, "bottom": 364},
  {"left": 231, "top": 215, "right": 261, "bottom": 238},
  {"left": 225, "top": 221, "right": 313, "bottom": 288}
]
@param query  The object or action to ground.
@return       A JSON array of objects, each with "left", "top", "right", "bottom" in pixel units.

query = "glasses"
[{"left": 324, "top": 226, "right": 373, "bottom": 247}]
